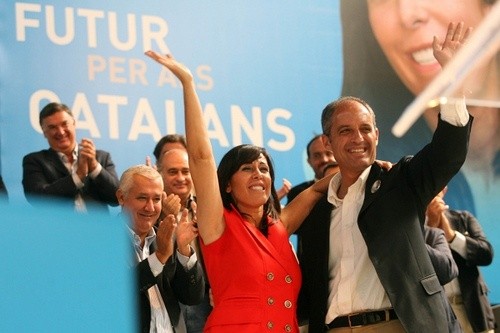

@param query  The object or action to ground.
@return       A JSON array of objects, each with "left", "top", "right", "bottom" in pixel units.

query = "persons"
[
  {"left": 421, "top": 185, "right": 500, "bottom": 333},
  {"left": 266, "top": 132, "right": 341, "bottom": 219},
  {"left": 21, "top": 102, "right": 119, "bottom": 215},
  {"left": 285, "top": 22, "right": 474, "bottom": 333},
  {"left": 144, "top": 48, "right": 399, "bottom": 333},
  {"left": 116, "top": 135, "right": 213, "bottom": 333},
  {"left": 339, "top": 0, "right": 500, "bottom": 306}
]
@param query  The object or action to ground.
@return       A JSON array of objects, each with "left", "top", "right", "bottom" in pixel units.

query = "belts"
[{"left": 327, "top": 310, "right": 398, "bottom": 329}]
